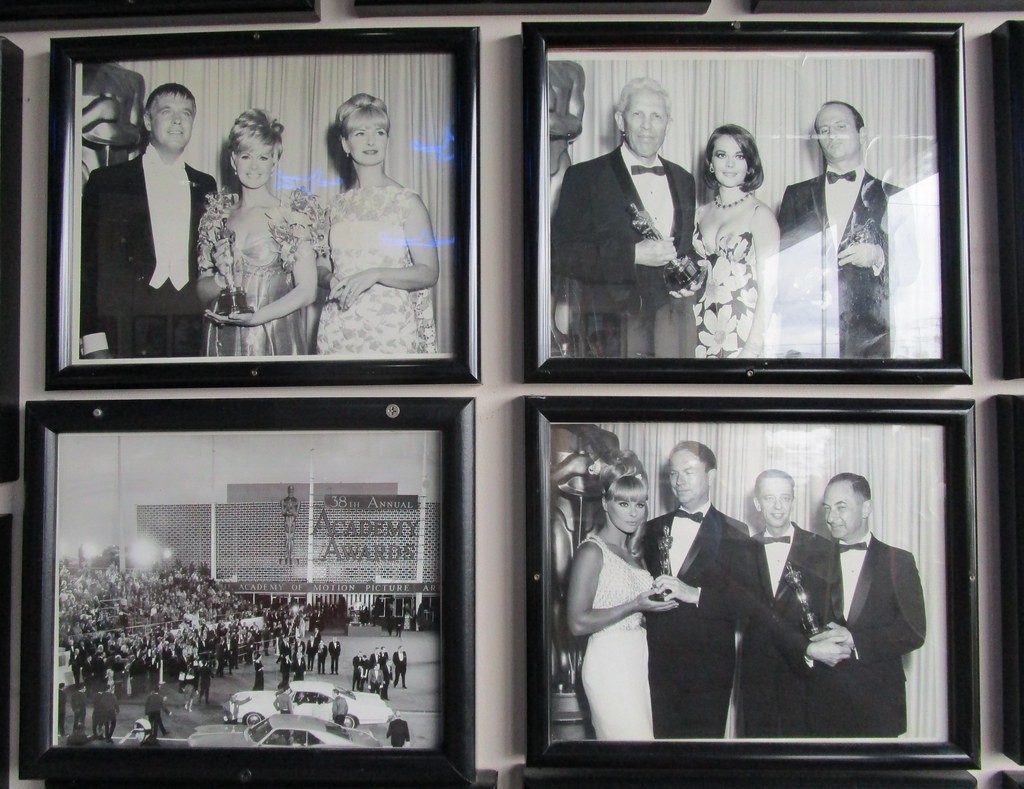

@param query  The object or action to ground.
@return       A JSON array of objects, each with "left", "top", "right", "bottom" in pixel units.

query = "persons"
[
  {"left": 776, "top": 473, "right": 925, "bottom": 739},
  {"left": 78, "top": 82, "right": 217, "bottom": 361},
  {"left": 386, "top": 711, "right": 410, "bottom": 748},
  {"left": 58, "top": 683, "right": 119, "bottom": 742},
  {"left": 695, "top": 124, "right": 781, "bottom": 357},
  {"left": 281, "top": 486, "right": 301, "bottom": 557},
  {"left": 145, "top": 686, "right": 172, "bottom": 737},
  {"left": 316, "top": 93, "right": 438, "bottom": 355},
  {"left": 778, "top": 102, "right": 921, "bottom": 359},
  {"left": 331, "top": 689, "right": 348, "bottom": 724},
  {"left": 642, "top": 440, "right": 751, "bottom": 739},
  {"left": 252, "top": 654, "right": 265, "bottom": 692},
  {"left": 361, "top": 602, "right": 417, "bottom": 637},
  {"left": 739, "top": 470, "right": 832, "bottom": 739},
  {"left": 59, "top": 561, "right": 341, "bottom": 712},
  {"left": 197, "top": 108, "right": 323, "bottom": 358},
  {"left": 79, "top": 544, "right": 84, "bottom": 566},
  {"left": 273, "top": 686, "right": 294, "bottom": 714},
  {"left": 353, "top": 646, "right": 407, "bottom": 700},
  {"left": 567, "top": 450, "right": 679, "bottom": 741},
  {"left": 197, "top": 660, "right": 214, "bottom": 704},
  {"left": 553, "top": 76, "right": 698, "bottom": 357}
]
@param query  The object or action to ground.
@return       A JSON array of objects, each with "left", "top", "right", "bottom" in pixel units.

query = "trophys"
[
  {"left": 217, "top": 219, "right": 253, "bottom": 313},
  {"left": 629, "top": 202, "right": 699, "bottom": 289},
  {"left": 786, "top": 561, "right": 821, "bottom": 637},
  {"left": 657, "top": 526, "right": 672, "bottom": 601}
]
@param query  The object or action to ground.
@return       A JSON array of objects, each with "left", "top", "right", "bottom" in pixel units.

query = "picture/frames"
[{"left": 0, "top": 1, "right": 1024, "bottom": 789}]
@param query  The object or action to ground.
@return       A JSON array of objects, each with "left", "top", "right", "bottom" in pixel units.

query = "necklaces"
[{"left": 713, "top": 193, "right": 752, "bottom": 207}]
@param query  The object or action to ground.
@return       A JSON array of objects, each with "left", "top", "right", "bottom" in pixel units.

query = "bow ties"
[
  {"left": 763, "top": 536, "right": 791, "bottom": 544},
  {"left": 825, "top": 170, "right": 856, "bottom": 184},
  {"left": 675, "top": 509, "right": 703, "bottom": 523},
  {"left": 839, "top": 542, "right": 868, "bottom": 553},
  {"left": 631, "top": 165, "right": 666, "bottom": 176}
]
